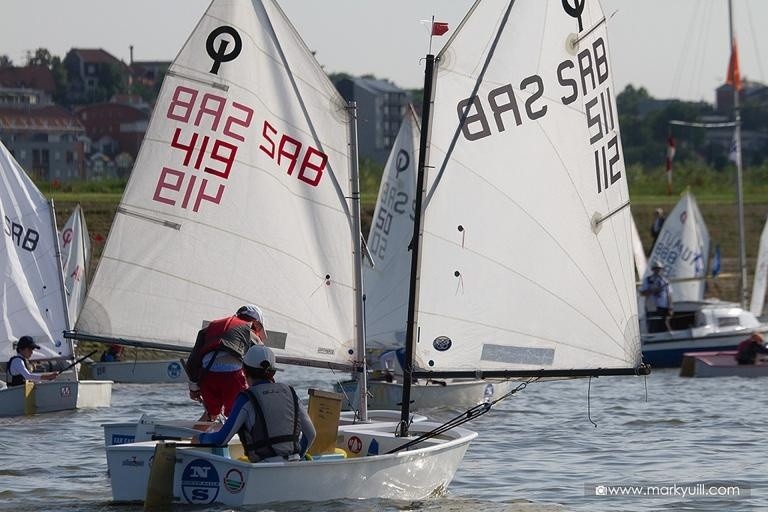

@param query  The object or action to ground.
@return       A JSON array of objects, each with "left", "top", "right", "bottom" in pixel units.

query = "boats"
[{"left": 62, "top": 0, "right": 653, "bottom": 502}]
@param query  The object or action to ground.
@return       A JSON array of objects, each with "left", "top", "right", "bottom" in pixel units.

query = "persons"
[
  {"left": 191, "top": 345, "right": 317, "bottom": 463},
  {"left": 379, "top": 347, "right": 407, "bottom": 384},
  {"left": 643, "top": 260, "right": 674, "bottom": 333},
  {"left": 189, "top": 305, "right": 268, "bottom": 431},
  {"left": 101, "top": 343, "right": 125, "bottom": 363},
  {"left": 649, "top": 207, "right": 665, "bottom": 236},
  {"left": 736, "top": 331, "right": 766, "bottom": 364},
  {"left": 6, "top": 336, "right": 58, "bottom": 387}
]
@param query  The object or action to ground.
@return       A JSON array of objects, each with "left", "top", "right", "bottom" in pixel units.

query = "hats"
[
  {"left": 651, "top": 260, "right": 664, "bottom": 270},
  {"left": 236, "top": 304, "right": 267, "bottom": 340},
  {"left": 243, "top": 344, "right": 284, "bottom": 372},
  {"left": 18, "top": 336, "right": 40, "bottom": 350}
]
